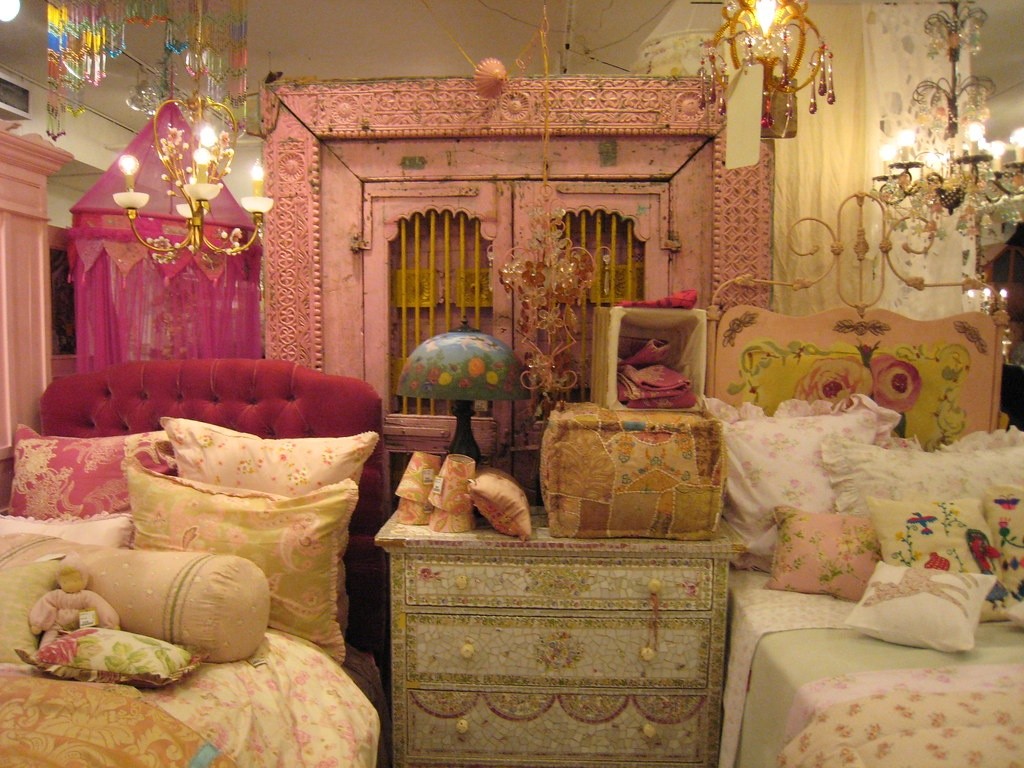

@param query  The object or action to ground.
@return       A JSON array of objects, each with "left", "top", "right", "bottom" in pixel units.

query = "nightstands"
[{"left": 371, "top": 515, "right": 743, "bottom": 768}]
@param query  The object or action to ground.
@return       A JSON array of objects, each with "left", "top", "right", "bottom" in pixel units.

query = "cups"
[{"left": 394, "top": 451, "right": 476, "bottom": 533}]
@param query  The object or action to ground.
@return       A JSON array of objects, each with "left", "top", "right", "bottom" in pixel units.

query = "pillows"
[
  {"left": 159, "top": 415, "right": 378, "bottom": 639},
  {"left": 0, "top": 559, "right": 59, "bottom": 663},
  {"left": 0, "top": 532, "right": 271, "bottom": 664},
  {"left": 876, "top": 498, "right": 1008, "bottom": 623},
  {"left": 844, "top": 560, "right": 998, "bottom": 651},
  {"left": 984, "top": 485, "right": 1024, "bottom": 626},
  {"left": 704, "top": 392, "right": 901, "bottom": 570},
  {"left": 821, "top": 425, "right": 1024, "bottom": 530},
  {"left": 10, "top": 423, "right": 175, "bottom": 519},
  {"left": 473, "top": 469, "right": 532, "bottom": 539},
  {"left": 119, "top": 457, "right": 358, "bottom": 662},
  {"left": 770, "top": 506, "right": 880, "bottom": 600},
  {"left": 14, "top": 627, "right": 204, "bottom": 689},
  {"left": 0, "top": 511, "right": 134, "bottom": 544}
]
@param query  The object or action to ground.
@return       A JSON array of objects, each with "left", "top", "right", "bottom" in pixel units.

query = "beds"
[
  {"left": 0, "top": 352, "right": 382, "bottom": 768},
  {"left": 707, "top": 192, "right": 1024, "bottom": 768}
]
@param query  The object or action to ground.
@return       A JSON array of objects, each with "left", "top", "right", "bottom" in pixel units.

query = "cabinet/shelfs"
[
  {"left": 0, "top": 128, "right": 74, "bottom": 515},
  {"left": 261, "top": 71, "right": 728, "bottom": 499}
]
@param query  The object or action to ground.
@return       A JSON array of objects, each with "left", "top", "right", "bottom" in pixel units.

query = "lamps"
[
  {"left": 694, "top": 0, "right": 836, "bottom": 139},
  {"left": 114, "top": 0, "right": 274, "bottom": 266},
  {"left": 959, "top": 257, "right": 1013, "bottom": 363},
  {"left": 863, "top": 1, "right": 1024, "bottom": 246},
  {"left": 397, "top": 316, "right": 524, "bottom": 463}
]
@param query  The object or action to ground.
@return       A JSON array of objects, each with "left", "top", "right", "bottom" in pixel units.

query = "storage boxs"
[{"left": 591, "top": 305, "right": 706, "bottom": 412}]
